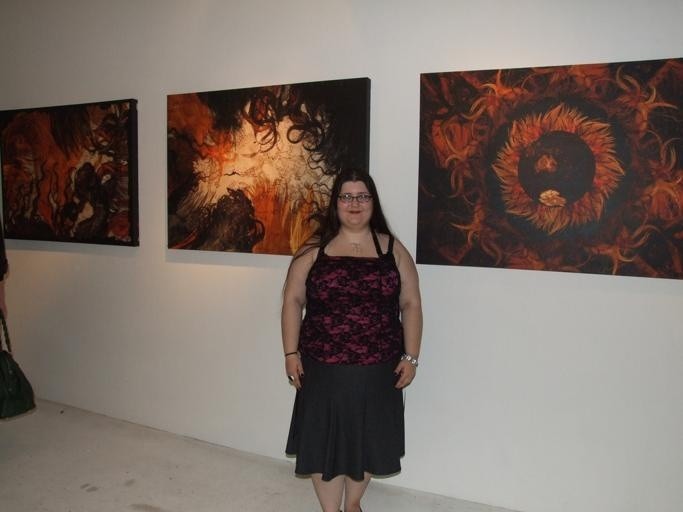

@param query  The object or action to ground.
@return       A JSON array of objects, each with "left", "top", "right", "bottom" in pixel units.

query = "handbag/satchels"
[{"left": 0, "top": 308, "right": 35, "bottom": 419}]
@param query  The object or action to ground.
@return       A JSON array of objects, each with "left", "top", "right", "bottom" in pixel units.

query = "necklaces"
[{"left": 341, "top": 228, "right": 369, "bottom": 254}]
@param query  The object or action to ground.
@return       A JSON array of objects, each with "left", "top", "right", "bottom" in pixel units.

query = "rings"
[{"left": 288, "top": 375, "right": 296, "bottom": 383}]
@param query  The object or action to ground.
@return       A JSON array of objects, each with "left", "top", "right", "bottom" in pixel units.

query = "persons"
[
  {"left": 279, "top": 166, "right": 424, "bottom": 512},
  {"left": 1, "top": 218, "right": 11, "bottom": 322}
]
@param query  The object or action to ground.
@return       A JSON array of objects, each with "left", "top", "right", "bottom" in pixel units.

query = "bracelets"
[
  {"left": 283, "top": 351, "right": 297, "bottom": 357},
  {"left": 400, "top": 353, "right": 420, "bottom": 369}
]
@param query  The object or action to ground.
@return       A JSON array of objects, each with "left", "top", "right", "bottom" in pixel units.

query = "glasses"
[{"left": 337, "top": 192, "right": 372, "bottom": 203}]
